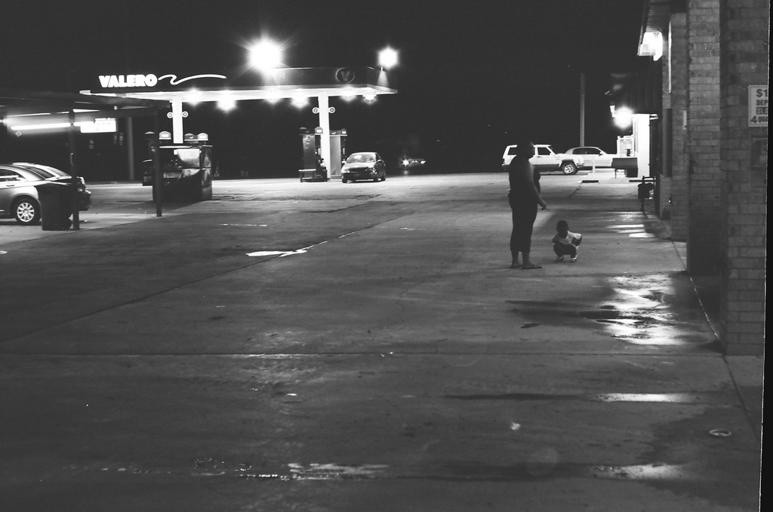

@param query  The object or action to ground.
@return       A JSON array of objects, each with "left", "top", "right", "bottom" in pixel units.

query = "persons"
[
  {"left": 507, "top": 137, "right": 548, "bottom": 269},
  {"left": 553, "top": 222, "right": 583, "bottom": 262}
]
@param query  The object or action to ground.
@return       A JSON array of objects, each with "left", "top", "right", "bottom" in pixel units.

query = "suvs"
[{"left": 501, "top": 143, "right": 584, "bottom": 175}]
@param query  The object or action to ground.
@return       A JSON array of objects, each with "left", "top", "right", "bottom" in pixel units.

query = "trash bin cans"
[{"left": 34, "top": 182, "right": 73, "bottom": 231}]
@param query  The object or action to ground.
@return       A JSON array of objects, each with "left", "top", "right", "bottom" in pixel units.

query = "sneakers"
[{"left": 556, "top": 256, "right": 578, "bottom": 262}]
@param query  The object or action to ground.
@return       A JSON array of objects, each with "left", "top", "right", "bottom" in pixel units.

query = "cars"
[
  {"left": 339, "top": 151, "right": 386, "bottom": 184},
  {"left": 564, "top": 146, "right": 625, "bottom": 169},
  {"left": 0, "top": 160, "right": 91, "bottom": 226},
  {"left": 397, "top": 152, "right": 431, "bottom": 170},
  {"left": 152, "top": 145, "right": 215, "bottom": 203}
]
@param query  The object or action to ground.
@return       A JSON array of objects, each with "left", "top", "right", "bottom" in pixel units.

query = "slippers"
[{"left": 513, "top": 261, "right": 541, "bottom": 268}]
